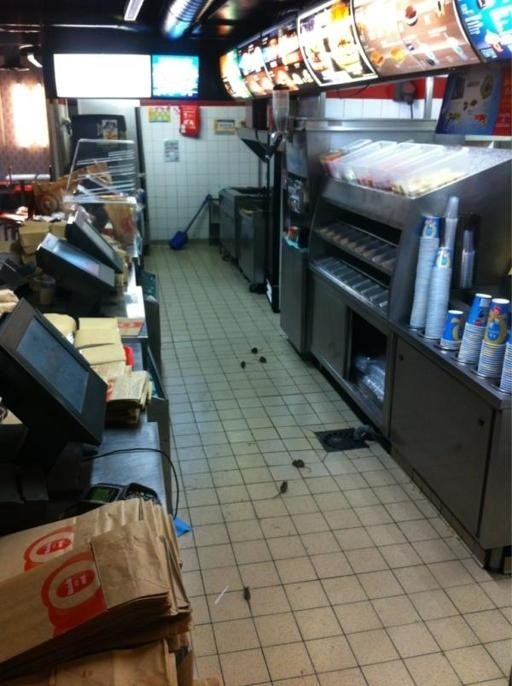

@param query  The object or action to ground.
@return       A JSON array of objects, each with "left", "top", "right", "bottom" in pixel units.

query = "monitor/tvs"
[
  {"left": 81, "top": 173, "right": 118, "bottom": 197},
  {"left": 0, "top": 297, "right": 107, "bottom": 458},
  {"left": 73, "top": 185, "right": 110, "bottom": 219},
  {"left": 37, "top": 232, "right": 117, "bottom": 300},
  {"left": 64, "top": 210, "right": 123, "bottom": 273}
]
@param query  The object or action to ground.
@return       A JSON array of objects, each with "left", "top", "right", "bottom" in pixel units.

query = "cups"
[{"left": 410, "top": 216, "right": 512, "bottom": 394}]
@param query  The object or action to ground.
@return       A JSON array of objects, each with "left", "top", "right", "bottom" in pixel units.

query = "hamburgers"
[{"left": 390, "top": 48, "right": 406, "bottom": 65}]
